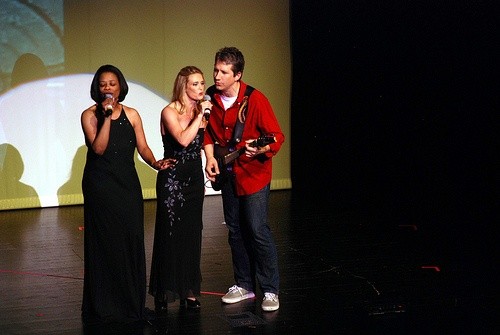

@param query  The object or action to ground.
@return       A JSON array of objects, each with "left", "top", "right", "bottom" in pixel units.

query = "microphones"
[
  {"left": 104, "top": 94, "right": 113, "bottom": 114},
  {"left": 203, "top": 95, "right": 211, "bottom": 121}
]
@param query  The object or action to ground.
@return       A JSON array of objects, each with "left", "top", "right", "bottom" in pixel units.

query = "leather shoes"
[
  {"left": 180, "top": 298, "right": 200, "bottom": 308},
  {"left": 155, "top": 298, "right": 168, "bottom": 310}
]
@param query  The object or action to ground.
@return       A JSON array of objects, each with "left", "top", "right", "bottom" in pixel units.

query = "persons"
[
  {"left": 81, "top": 64, "right": 176, "bottom": 325},
  {"left": 149, "top": 66, "right": 213, "bottom": 311},
  {"left": 202, "top": 47, "right": 285, "bottom": 311}
]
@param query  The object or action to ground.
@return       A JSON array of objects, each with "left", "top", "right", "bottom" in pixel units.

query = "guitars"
[{"left": 211, "top": 133, "right": 278, "bottom": 191}]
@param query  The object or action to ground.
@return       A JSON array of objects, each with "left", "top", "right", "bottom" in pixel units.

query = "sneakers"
[
  {"left": 262, "top": 292, "right": 279, "bottom": 311},
  {"left": 222, "top": 285, "right": 255, "bottom": 303}
]
